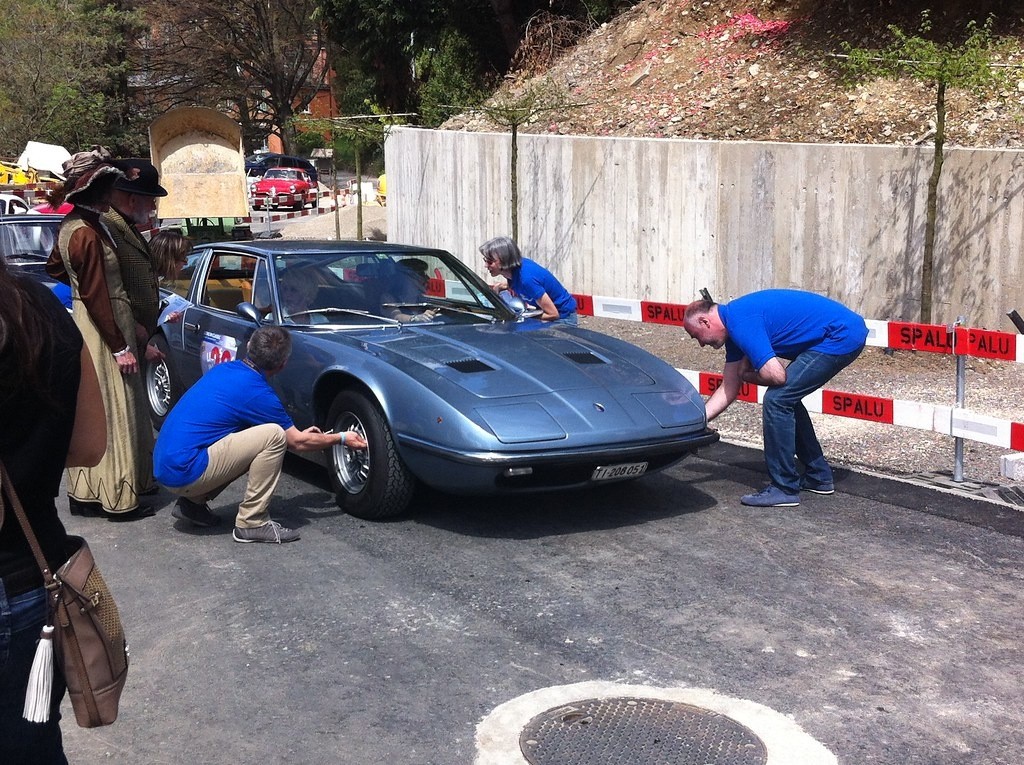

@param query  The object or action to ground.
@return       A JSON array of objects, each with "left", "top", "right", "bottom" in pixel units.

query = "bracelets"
[
  {"left": 339, "top": 432, "right": 346, "bottom": 445},
  {"left": 411, "top": 315, "right": 417, "bottom": 322}
]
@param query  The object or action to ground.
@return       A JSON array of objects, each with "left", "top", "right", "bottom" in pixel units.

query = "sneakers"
[
  {"left": 800, "top": 473, "right": 834, "bottom": 494},
  {"left": 233, "top": 520, "right": 301, "bottom": 544},
  {"left": 740, "top": 482, "right": 801, "bottom": 506},
  {"left": 172, "top": 497, "right": 222, "bottom": 527}
]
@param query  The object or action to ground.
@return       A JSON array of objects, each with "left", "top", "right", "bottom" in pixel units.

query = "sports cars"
[{"left": 145, "top": 237, "right": 722, "bottom": 520}]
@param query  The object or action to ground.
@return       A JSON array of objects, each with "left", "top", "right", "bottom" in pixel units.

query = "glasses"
[
  {"left": 181, "top": 257, "right": 188, "bottom": 263},
  {"left": 483, "top": 256, "right": 499, "bottom": 263}
]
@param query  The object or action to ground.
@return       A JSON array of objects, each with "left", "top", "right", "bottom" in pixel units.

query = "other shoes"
[
  {"left": 69, "top": 496, "right": 105, "bottom": 517},
  {"left": 98, "top": 504, "right": 155, "bottom": 518}
]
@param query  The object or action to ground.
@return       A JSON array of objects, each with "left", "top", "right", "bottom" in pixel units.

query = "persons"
[
  {"left": 479, "top": 237, "right": 577, "bottom": 326},
  {"left": 379, "top": 257, "right": 435, "bottom": 323},
  {"left": 362, "top": 174, "right": 386, "bottom": 207},
  {"left": 265, "top": 263, "right": 328, "bottom": 325},
  {"left": 150, "top": 325, "right": 367, "bottom": 543},
  {"left": 44, "top": 155, "right": 190, "bottom": 520},
  {"left": 684, "top": 289, "right": 867, "bottom": 506},
  {"left": 0, "top": 260, "right": 84, "bottom": 765}
]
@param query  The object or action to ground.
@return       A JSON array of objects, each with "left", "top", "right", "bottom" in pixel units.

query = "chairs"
[
  {"left": 207, "top": 289, "right": 252, "bottom": 311},
  {"left": 363, "top": 277, "right": 387, "bottom": 316}
]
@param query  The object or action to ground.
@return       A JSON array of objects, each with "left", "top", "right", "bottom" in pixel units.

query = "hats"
[
  {"left": 114, "top": 158, "right": 168, "bottom": 196},
  {"left": 46, "top": 144, "right": 141, "bottom": 209}
]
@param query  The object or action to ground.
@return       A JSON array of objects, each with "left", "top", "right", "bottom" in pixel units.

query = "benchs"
[{"left": 165, "top": 278, "right": 361, "bottom": 313}]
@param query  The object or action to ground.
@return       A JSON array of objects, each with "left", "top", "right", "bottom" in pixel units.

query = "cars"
[
  {"left": 250, "top": 167, "right": 318, "bottom": 212},
  {"left": 243, "top": 154, "right": 319, "bottom": 183},
  {"left": 0, "top": 158, "right": 71, "bottom": 274}
]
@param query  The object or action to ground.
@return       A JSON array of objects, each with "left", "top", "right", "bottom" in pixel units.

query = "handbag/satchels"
[{"left": 23, "top": 535, "right": 130, "bottom": 728}]
